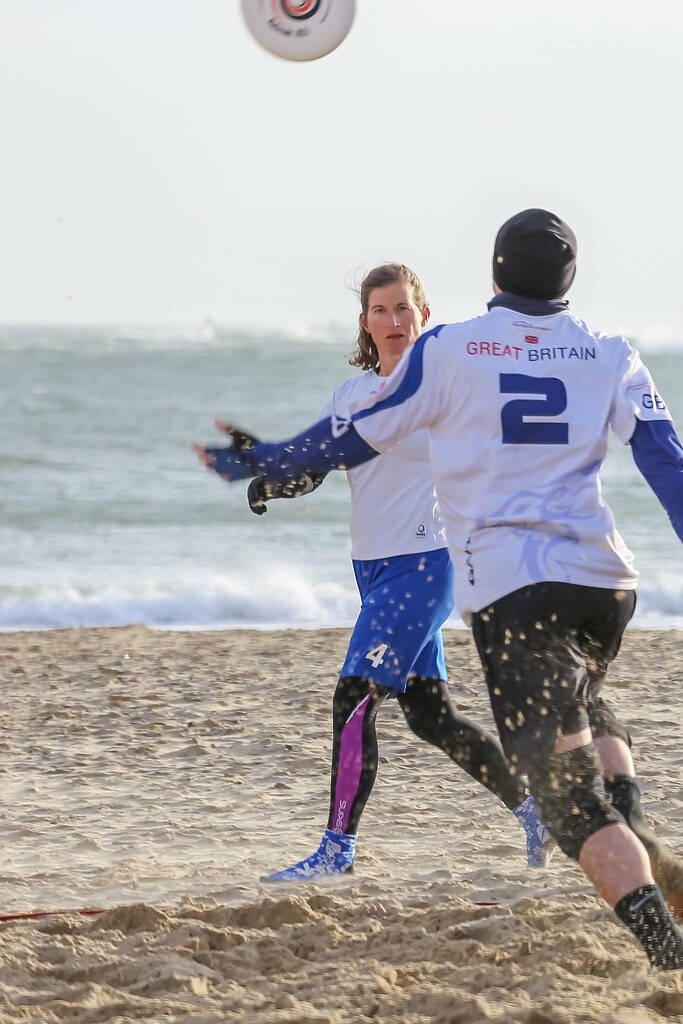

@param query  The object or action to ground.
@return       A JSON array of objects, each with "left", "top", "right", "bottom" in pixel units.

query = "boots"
[
  {"left": 512, "top": 796, "right": 559, "bottom": 869},
  {"left": 260, "top": 827, "right": 356, "bottom": 885}
]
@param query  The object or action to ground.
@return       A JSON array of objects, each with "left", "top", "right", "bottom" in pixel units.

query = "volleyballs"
[{"left": 237, "top": 1, "right": 358, "bottom": 62}]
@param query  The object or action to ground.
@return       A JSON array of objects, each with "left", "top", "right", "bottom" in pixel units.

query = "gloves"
[{"left": 247, "top": 468, "right": 323, "bottom": 515}]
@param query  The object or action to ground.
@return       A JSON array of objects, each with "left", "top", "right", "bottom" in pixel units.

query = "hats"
[{"left": 493, "top": 209, "right": 577, "bottom": 299}]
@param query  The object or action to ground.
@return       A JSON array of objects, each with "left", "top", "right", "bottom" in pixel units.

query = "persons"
[{"left": 189, "top": 206, "right": 683, "bottom": 971}]
